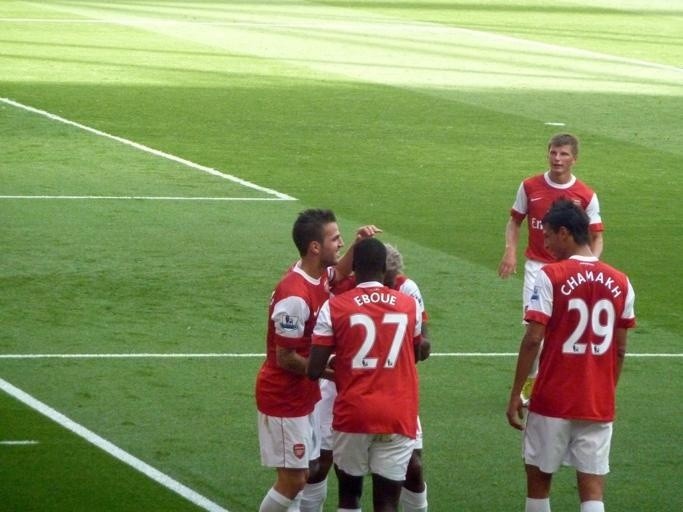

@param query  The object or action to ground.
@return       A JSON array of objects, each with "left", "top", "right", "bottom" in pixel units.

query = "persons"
[
  {"left": 497, "top": 132, "right": 604, "bottom": 407},
  {"left": 504, "top": 192, "right": 636, "bottom": 512},
  {"left": 254, "top": 207, "right": 383, "bottom": 512}
]
